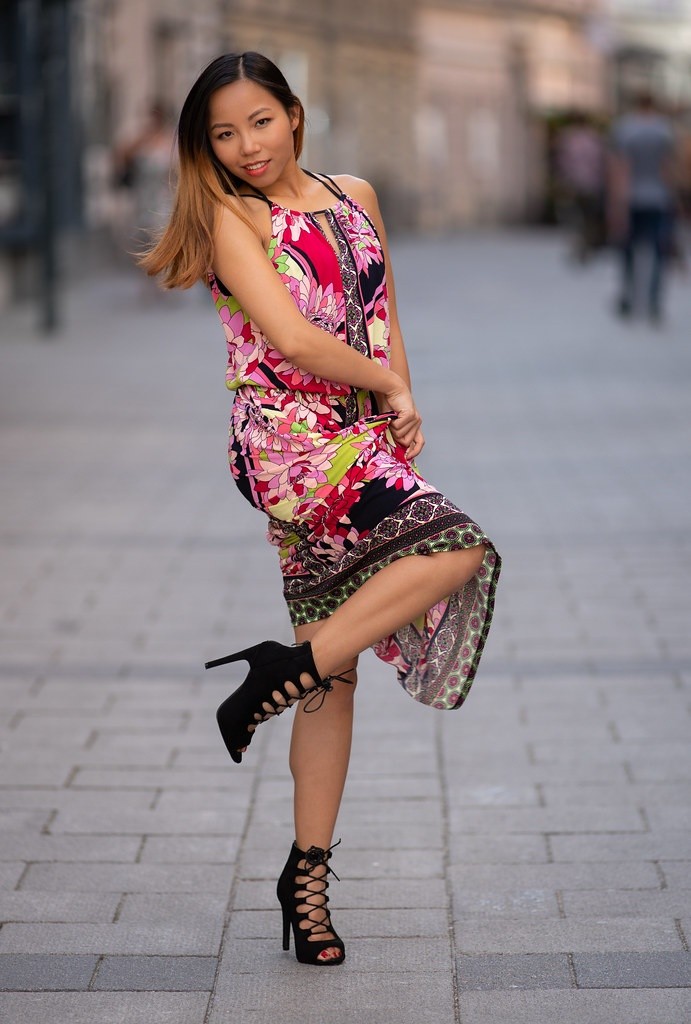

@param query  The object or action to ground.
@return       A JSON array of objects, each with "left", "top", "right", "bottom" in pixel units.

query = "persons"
[
  {"left": 128, "top": 52, "right": 502, "bottom": 967},
  {"left": 550, "top": 87, "right": 691, "bottom": 327}
]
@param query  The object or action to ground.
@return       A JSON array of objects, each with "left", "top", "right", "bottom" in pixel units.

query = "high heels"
[
  {"left": 276, "top": 839, "right": 345, "bottom": 966},
  {"left": 204, "top": 640, "right": 356, "bottom": 763}
]
